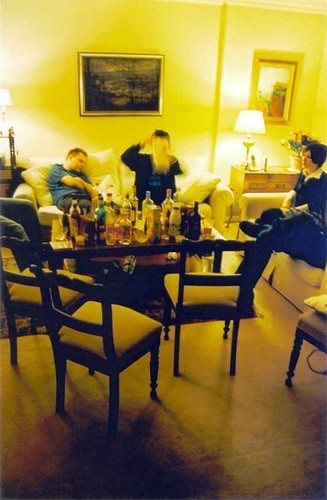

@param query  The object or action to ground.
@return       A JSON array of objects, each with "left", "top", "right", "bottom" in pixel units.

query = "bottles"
[
  {"left": 84, "top": 205, "right": 95, "bottom": 241},
  {"left": 97, "top": 197, "right": 107, "bottom": 233},
  {"left": 69, "top": 203, "right": 81, "bottom": 237},
  {"left": 186, "top": 201, "right": 201, "bottom": 241},
  {"left": 167, "top": 205, "right": 182, "bottom": 237},
  {"left": 61, "top": 204, "right": 70, "bottom": 240},
  {"left": 118, "top": 212, "right": 133, "bottom": 246},
  {"left": 104, "top": 192, "right": 117, "bottom": 218},
  {"left": 105, "top": 220, "right": 121, "bottom": 245},
  {"left": 146, "top": 205, "right": 161, "bottom": 242},
  {"left": 128, "top": 184, "right": 139, "bottom": 220},
  {"left": 121, "top": 194, "right": 131, "bottom": 219},
  {"left": 142, "top": 190, "right": 155, "bottom": 225},
  {"left": 161, "top": 188, "right": 174, "bottom": 225},
  {"left": 97, "top": 193, "right": 103, "bottom": 197}
]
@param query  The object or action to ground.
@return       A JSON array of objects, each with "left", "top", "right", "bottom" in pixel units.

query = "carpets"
[{"left": 0, "top": 243, "right": 257, "bottom": 342}]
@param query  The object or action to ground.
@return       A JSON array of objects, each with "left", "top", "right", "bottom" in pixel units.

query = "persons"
[
  {"left": 233, "top": 208, "right": 326, "bottom": 318},
  {"left": 255, "top": 143, "right": 327, "bottom": 225},
  {"left": 48, "top": 148, "right": 108, "bottom": 215},
  {"left": 0, "top": 197, "right": 47, "bottom": 273},
  {"left": 121, "top": 130, "right": 182, "bottom": 210}
]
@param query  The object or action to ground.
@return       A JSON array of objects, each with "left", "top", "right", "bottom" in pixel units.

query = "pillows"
[
  {"left": 20, "top": 165, "right": 53, "bottom": 205},
  {"left": 174, "top": 174, "right": 220, "bottom": 202}
]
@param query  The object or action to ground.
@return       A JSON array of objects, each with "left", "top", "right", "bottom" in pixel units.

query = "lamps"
[{"left": 234, "top": 110, "right": 266, "bottom": 167}]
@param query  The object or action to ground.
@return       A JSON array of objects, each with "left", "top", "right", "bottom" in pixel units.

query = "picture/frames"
[
  {"left": 250, "top": 56, "right": 297, "bottom": 122},
  {"left": 79, "top": 51, "right": 164, "bottom": 117}
]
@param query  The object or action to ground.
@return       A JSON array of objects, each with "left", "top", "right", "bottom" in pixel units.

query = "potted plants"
[{"left": 280, "top": 128, "right": 320, "bottom": 169}]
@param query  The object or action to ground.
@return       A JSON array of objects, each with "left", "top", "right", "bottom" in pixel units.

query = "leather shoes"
[{"left": 240, "top": 220, "right": 266, "bottom": 238}]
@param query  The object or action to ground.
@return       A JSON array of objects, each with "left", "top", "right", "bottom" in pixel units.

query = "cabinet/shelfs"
[{"left": 225, "top": 164, "right": 300, "bottom": 240}]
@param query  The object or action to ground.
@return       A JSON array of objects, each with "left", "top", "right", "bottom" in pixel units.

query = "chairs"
[
  {"left": 164, "top": 238, "right": 255, "bottom": 377},
  {"left": 30, "top": 265, "right": 161, "bottom": 436},
  {"left": 0, "top": 252, "right": 96, "bottom": 366},
  {"left": 284, "top": 299, "right": 327, "bottom": 387}
]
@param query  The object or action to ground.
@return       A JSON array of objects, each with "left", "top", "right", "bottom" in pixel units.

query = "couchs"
[
  {"left": 13, "top": 148, "right": 234, "bottom": 238},
  {"left": 235, "top": 184, "right": 327, "bottom": 314}
]
